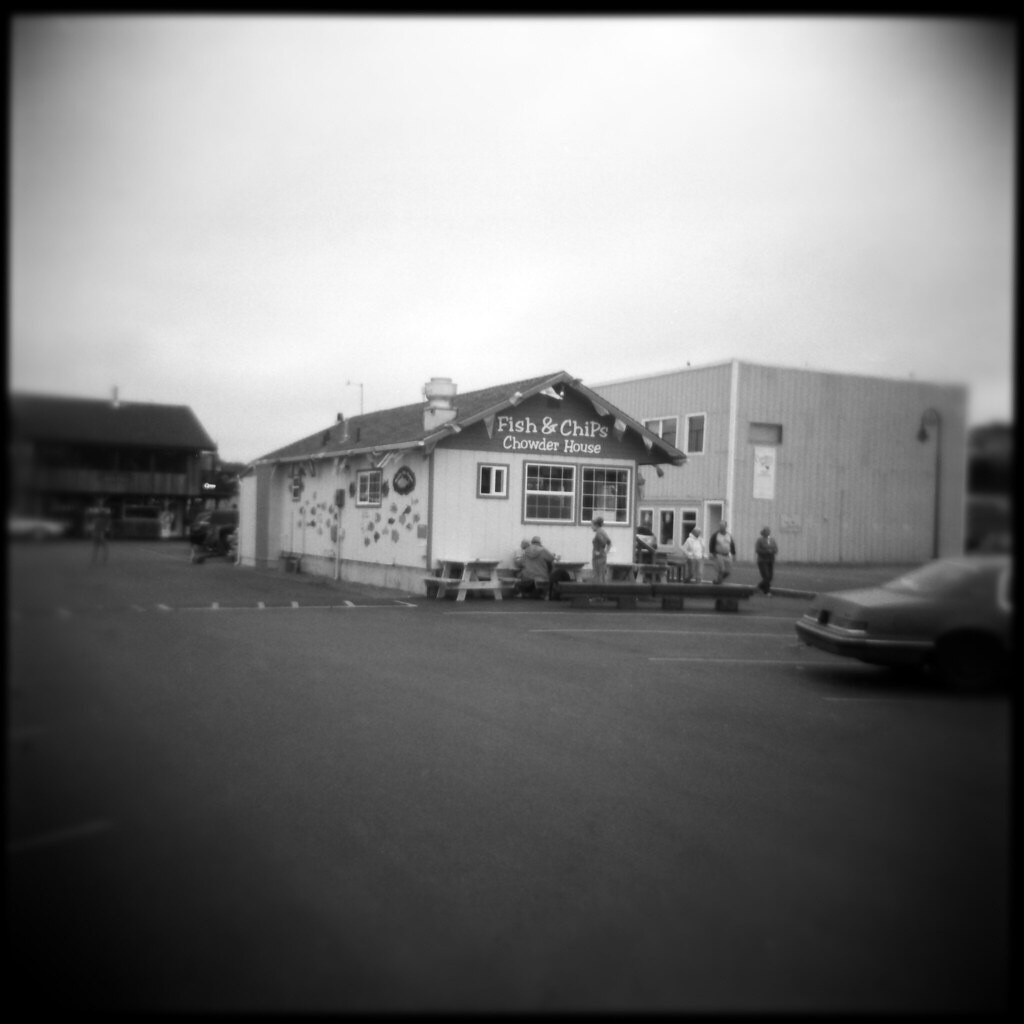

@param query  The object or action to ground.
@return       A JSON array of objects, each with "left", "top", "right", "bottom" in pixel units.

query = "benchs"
[
  {"left": 422, "top": 574, "right": 551, "bottom": 591},
  {"left": 555, "top": 582, "right": 757, "bottom": 613}
]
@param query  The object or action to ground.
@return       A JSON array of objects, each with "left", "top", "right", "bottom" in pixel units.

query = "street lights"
[{"left": 918, "top": 407, "right": 941, "bottom": 555}]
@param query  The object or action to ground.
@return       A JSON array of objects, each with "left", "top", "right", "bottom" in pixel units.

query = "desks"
[
  {"left": 436, "top": 556, "right": 504, "bottom": 601},
  {"left": 606, "top": 562, "right": 660, "bottom": 585},
  {"left": 518, "top": 560, "right": 590, "bottom": 582}
]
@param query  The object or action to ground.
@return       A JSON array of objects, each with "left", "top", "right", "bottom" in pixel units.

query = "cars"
[
  {"left": 185, "top": 510, "right": 239, "bottom": 560},
  {"left": 795, "top": 554, "right": 1012, "bottom": 669},
  {"left": 635, "top": 526, "right": 657, "bottom": 552}
]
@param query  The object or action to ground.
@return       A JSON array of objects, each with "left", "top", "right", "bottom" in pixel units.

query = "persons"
[
  {"left": 633, "top": 518, "right": 658, "bottom": 585},
  {"left": 681, "top": 526, "right": 705, "bottom": 585},
  {"left": 511, "top": 535, "right": 554, "bottom": 600},
  {"left": 755, "top": 527, "right": 779, "bottom": 597},
  {"left": 591, "top": 516, "right": 612, "bottom": 603},
  {"left": 708, "top": 519, "right": 736, "bottom": 585},
  {"left": 89, "top": 496, "right": 112, "bottom": 567}
]
